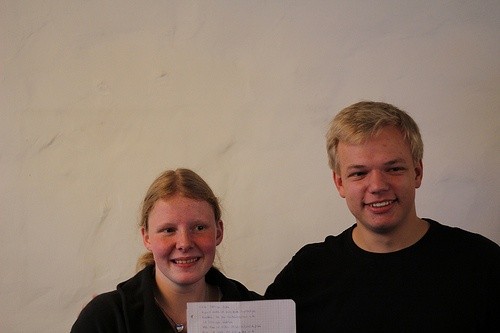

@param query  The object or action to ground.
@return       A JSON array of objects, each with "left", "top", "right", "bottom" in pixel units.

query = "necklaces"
[{"left": 155, "top": 282, "right": 210, "bottom": 332}]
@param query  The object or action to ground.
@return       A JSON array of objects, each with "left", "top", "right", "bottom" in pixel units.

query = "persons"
[
  {"left": 68, "top": 167, "right": 261, "bottom": 332},
  {"left": 264, "top": 101, "right": 499, "bottom": 333}
]
